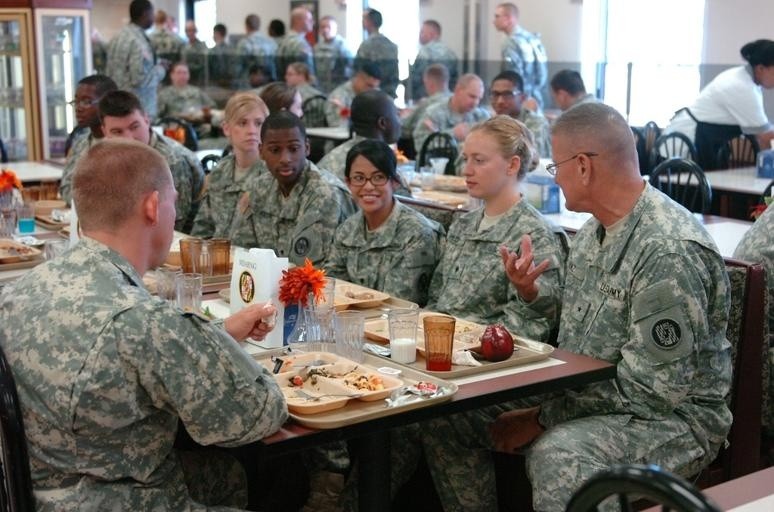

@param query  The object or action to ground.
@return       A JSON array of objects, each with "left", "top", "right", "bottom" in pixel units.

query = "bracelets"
[{"left": 535, "top": 405, "right": 547, "bottom": 431}]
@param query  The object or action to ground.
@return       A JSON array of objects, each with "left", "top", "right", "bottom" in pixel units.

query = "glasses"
[
  {"left": 346, "top": 171, "right": 392, "bottom": 186},
  {"left": 68, "top": 95, "right": 105, "bottom": 110},
  {"left": 488, "top": 89, "right": 520, "bottom": 100},
  {"left": 546, "top": 153, "right": 598, "bottom": 176}
]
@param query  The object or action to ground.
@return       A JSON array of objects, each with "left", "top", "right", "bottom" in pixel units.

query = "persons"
[
  {"left": 184, "top": 91, "right": 270, "bottom": 244},
  {"left": 728, "top": 198, "right": 773, "bottom": 435},
  {"left": 0, "top": 134, "right": 289, "bottom": 512},
  {"left": 320, "top": 138, "right": 447, "bottom": 307},
  {"left": 58, "top": 88, "right": 207, "bottom": 238},
  {"left": 228, "top": 109, "right": 356, "bottom": 275},
  {"left": 414, "top": 99, "right": 732, "bottom": 511},
  {"left": 62, "top": 74, "right": 120, "bottom": 200},
  {"left": 650, "top": 38, "right": 773, "bottom": 217},
  {"left": 95, "top": 0, "right": 625, "bottom": 206},
  {"left": 292, "top": 113, "right": 569, "bottom": 512}
]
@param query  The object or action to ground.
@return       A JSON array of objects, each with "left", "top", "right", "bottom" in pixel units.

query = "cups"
[
  {"left": 388, "top": 309, "right": 419, "bottom": 364},
  {"left": 398, "top": 156, "right": 450, "bottom": 192},
  {"left": 301, "top": 274, "right": 365, "bottom": 365},
  {"left": 421, "top": 315, "right": 456, "bottom": 372},
  {"left": 1, "top": 191, "right": 69, "bottom": 264},
  {"left": 164, "top": 121, "right": 186, "bottom": 144},
  {"left": 154, "top": 236, "right": 231, "bottom": 313}
]
